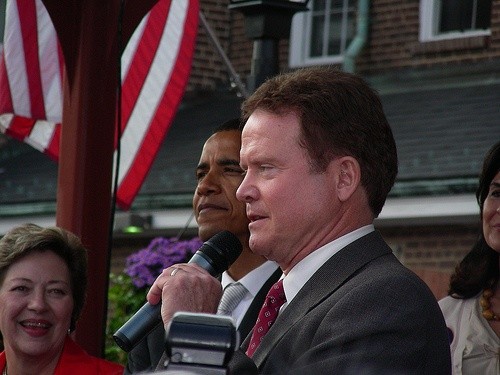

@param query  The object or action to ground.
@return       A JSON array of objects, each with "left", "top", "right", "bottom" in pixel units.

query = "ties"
[
  {"left": 248, "top": 279, "right": 287, "bottom": 359},
  {"left": 215, "top": 284, "right": 248, "bottom": 317}
]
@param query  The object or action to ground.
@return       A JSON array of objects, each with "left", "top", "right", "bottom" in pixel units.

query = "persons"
[
  {"left": 0, "top": 223, "right": 124, "bottom": 375},
  {"left": 436, "top": 142, "right": 500, "bottom": 375},
  {"left": 123, "top": 118, "right": 283, "bottom": 375},
  {"left": 146, "top": 66, "right": 452, "bottom": 375}
]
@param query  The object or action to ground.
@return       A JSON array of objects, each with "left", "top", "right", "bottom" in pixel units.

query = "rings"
[{"left": 172, "top": 267, "right": 182, "bottom": 276}]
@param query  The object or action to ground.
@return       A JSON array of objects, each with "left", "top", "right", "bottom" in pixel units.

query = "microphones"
[{"left": 112, "top": 229, "right": 243, "bottom": 353}]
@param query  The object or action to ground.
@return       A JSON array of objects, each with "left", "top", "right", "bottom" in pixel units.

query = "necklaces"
[{"left": 480, "top": 288, "right": 500, "bottom": 322}]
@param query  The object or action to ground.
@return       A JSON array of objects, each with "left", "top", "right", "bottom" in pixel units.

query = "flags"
[{"left": 0, "top": 0, "right": 199, "bottom": 209}]
